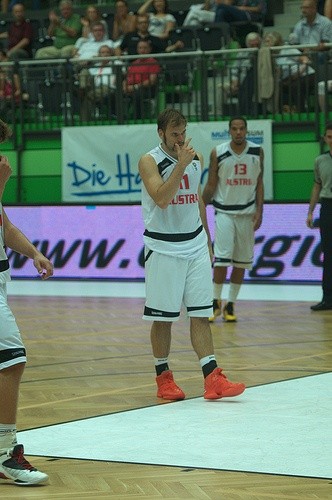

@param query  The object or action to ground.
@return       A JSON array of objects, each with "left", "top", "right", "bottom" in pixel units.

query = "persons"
[
  {"left": 0, "top": 120, "right": 55, "bottom": 487},
  {"left": 138, "top": 110, "right": 246, "bottom": 400},
  {"left": 0, "top": 0, "right": 332, "bottom": 124},
  {"left": 203, "top": 117, "right": 266, "bottom": 322},
  {"left": 306, "top": 124, "right": 332, "bottom": 310}
]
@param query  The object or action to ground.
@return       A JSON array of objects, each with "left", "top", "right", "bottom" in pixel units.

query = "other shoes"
[
  {"left": 209, "top": 298, "right": 222, "bottom": 322},
  {"left": 310, "top": 300, "right": 331, "bottom": 310},
  {"left": 224, "top": 301, "right": 237, "bottom": 322}
]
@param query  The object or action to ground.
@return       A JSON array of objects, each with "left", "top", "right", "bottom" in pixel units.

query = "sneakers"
[
  {"left": 204, "top": 368, "right": 245, "bottom": 398},
  {"left": 156, "top": 371, "right": 185, "bottom": 399},
  {"left": 0, "top": 444, "right": 48, "bottom": 485}
]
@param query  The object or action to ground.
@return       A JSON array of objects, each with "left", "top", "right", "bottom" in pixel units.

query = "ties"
[{"left": 98, "top": 66, "right": 102, "bottom": 75}]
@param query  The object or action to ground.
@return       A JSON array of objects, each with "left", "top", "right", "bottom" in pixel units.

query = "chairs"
[{"left": 0, "top": 0, "right": 264, "bottom": 116}]
